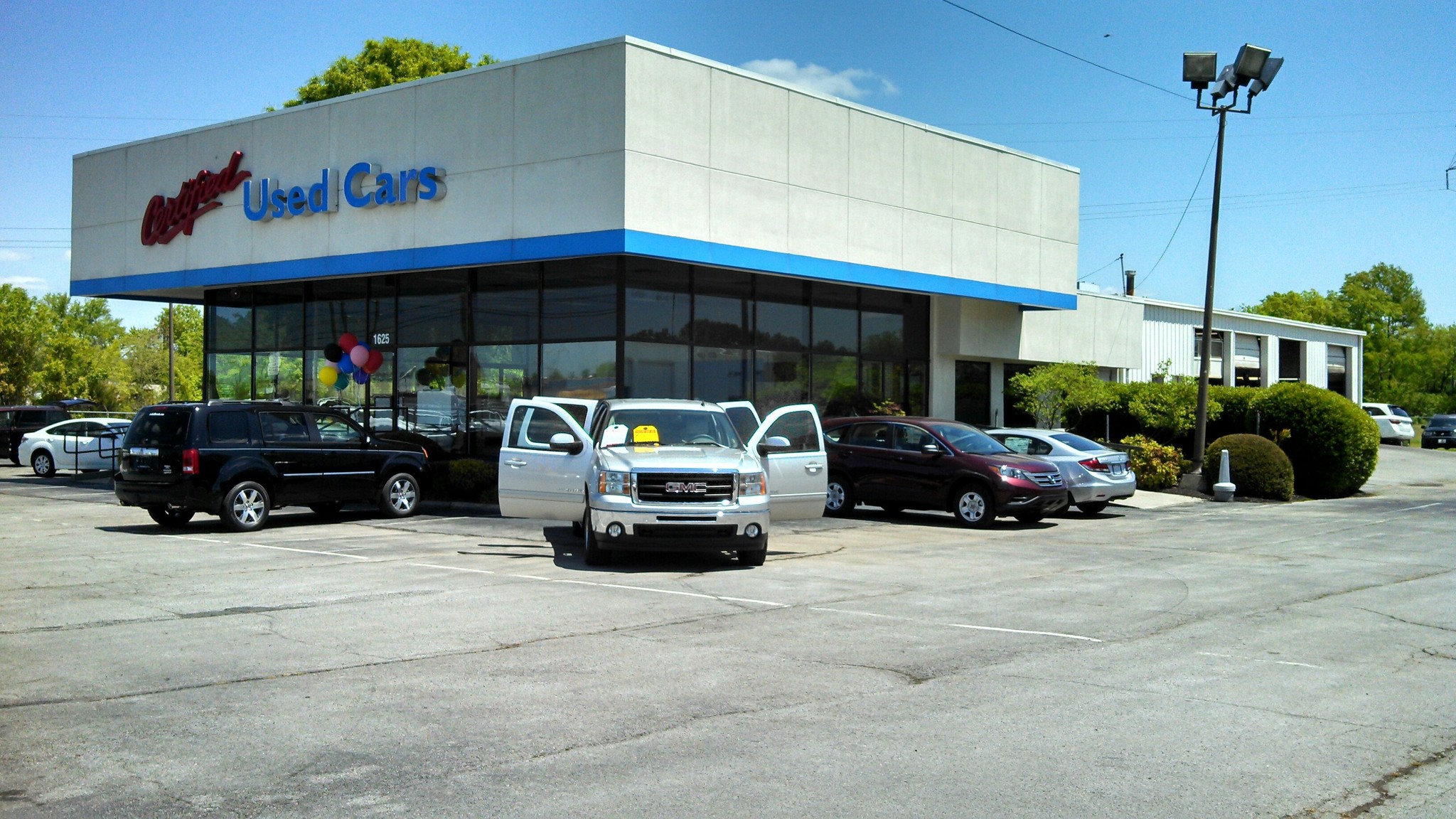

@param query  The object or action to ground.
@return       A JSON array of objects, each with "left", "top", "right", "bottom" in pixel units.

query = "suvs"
[
  {"left": 0, "top": 398, "right": 99, "bottom": 464},
  {"left": 804, "top": 416, "right": 1069, "bottom": 528},
  {"left": 498, "top": 395, "right": 829, "bottom": 564},
  {"left": 1362, "top": 402, "right": 1415, "bottom": 446},
  {"left": 114, "top": 399, "right": 432, "bottom": 533}
]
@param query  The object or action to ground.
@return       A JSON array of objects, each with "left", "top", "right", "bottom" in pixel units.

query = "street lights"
[{"left": 1182, "top": 43, "right": 1285, "bottom": 476}]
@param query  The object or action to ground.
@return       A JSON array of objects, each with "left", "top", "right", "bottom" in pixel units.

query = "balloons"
[
  {"left": 415, "top": 339, "right": 467, "bottom": 392},
  {"left": 318, "top": 332, "right": 384, "bottom": 393}
]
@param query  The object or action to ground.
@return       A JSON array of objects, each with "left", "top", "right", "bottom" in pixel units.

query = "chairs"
[
  {"left": 918, "top": 434, "right": 933, "bottom": 453},
  {"left": 281, "top": 423, "right": 312, "bottom": 442},
  {"left": 682, "top": 418, "right": 710, "bottom": 443}
]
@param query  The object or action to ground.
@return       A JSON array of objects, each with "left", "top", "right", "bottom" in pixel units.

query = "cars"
[
  {"left": 1421, "top": 414, "right": 1456, "bottom": 450},
  {"left": 949, "top": 428, "right": 1137, "bottom": 517},
  {"left": 18, "top": 418, "right": 132, "bottom": 477},
  {"left": 257, "top": 397, "right": 526, "bottom": 445}
]
